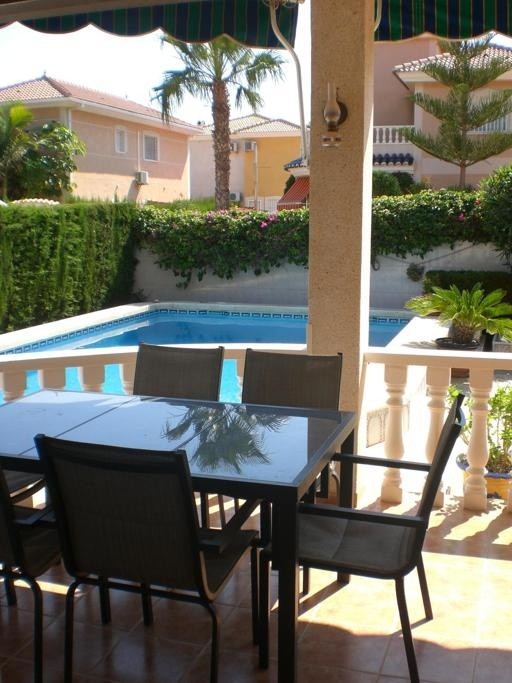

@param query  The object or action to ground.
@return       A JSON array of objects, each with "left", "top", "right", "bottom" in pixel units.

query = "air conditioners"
[
  {"left": 230, "top": 143, "right": 237, "bottom": 151},
  {"left": 244, "top": 142, "right": 256, "bottom": 152},
  {"left": 230, "top": 192, "right": 240, "bottom": 202},
  {"left": 134, "top": 172, "right": 148, "bottom": 185}
]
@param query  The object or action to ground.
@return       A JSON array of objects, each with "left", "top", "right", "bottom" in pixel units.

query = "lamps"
[{"left": 324, "top": 83, "right": 347, "bottom": 131}]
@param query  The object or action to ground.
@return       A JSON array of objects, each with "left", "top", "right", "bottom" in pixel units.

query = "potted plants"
[
  {"left": 445, "top": 383, "right": 512, "bottom": 499},
  {"left": 403, "top": 282, "right": 512, "bottom": 349}
]
